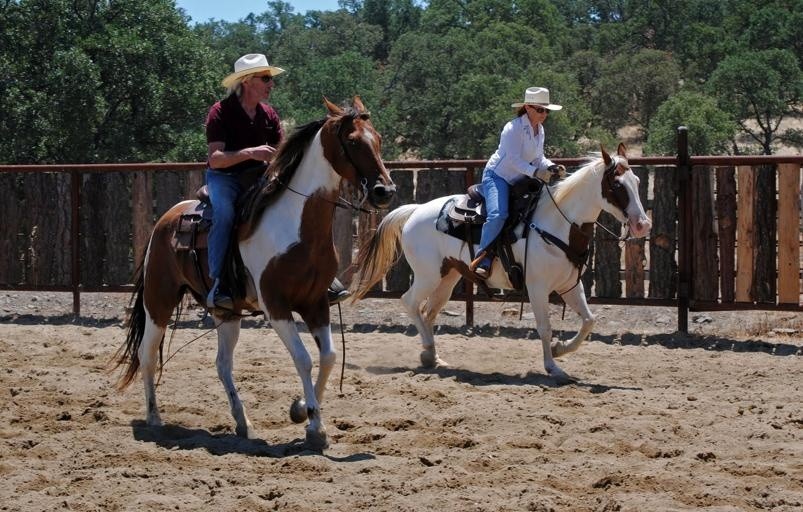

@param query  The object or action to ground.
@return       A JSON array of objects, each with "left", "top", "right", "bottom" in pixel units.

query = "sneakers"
[{"left": 475, "top": 266, "right": 490, "bottom": 279}]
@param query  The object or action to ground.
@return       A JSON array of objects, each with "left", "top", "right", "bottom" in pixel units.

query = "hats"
[
  {"left": 511, "top": 87, "right": 563, "bottom": 111},
  {"left": 221, "top": 53, "right": 286, "bottom": 88}
]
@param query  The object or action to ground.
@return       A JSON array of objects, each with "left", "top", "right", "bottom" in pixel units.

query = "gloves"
[
  {"left": 557, "top": 164, "right": 566, "bottom": 179},
  {"left": 536, "top": 169, "right": 555, "bottom": 184}
]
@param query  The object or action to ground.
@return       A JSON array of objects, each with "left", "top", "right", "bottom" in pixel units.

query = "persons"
[
  {"left": 466, "top": 86, "right": 570, "bottom": 279},
  {"left": 199, "top": 52, "right": 353, "bottom": 311}
]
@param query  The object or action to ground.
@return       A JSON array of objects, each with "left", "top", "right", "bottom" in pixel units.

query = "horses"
[
  {"left": 339, "top": 142, "right": 652, "bottom": 378},
  {"left": 107, "top": 94, "right": 396, "bottom": 448}
]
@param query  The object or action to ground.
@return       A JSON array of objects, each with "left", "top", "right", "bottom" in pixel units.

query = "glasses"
[
  {"left": 252, "top": 76, "right": 272, "bottom": 83},
  {"left": 529, "top": 105, "right": 550, "bottom": 113}
]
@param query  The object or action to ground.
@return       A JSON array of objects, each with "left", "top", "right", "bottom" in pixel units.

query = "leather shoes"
[
  {"left": 212, "top": 285, "right": 233, "bottom": 320},
  {"left": 328, "top": 289, "right": 351, "bottom": 304}
]
